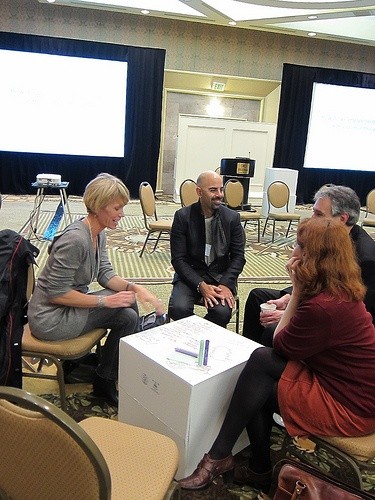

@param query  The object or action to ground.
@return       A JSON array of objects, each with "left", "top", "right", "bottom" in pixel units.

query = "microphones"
[{"left": 249, "top": 152, "right": 250, "bottom": 160}]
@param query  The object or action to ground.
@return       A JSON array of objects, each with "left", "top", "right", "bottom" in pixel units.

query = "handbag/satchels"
[
  {"left": 273, "top": 463, "right": 365, "bottom": 500},
  {"left": 57, "top": 352, "right": 97, "bottom": 383}
]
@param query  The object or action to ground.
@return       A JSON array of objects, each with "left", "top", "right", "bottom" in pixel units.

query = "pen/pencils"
[
  {"left": 175, "top": 348, "right": 198, "bottom": 358},
  {"left": 203, "top": 340, "right": 209, "bottom": 366},
  {"left": 198, "top": 340, "right": 204, "bottom": 365}
]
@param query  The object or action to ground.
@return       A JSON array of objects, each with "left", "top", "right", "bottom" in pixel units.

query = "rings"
[
  {"left": 291, "top": 270, "right": 295, "bottom": 275},
  {"left": 230, "top": 297, "right": 232, "bottom": 299}
]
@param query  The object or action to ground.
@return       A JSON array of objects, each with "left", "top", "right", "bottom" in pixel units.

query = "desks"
[
  {"left": 118, "top": 314, "right": 265, "bottom": 482},
  {"left": 262, "top": 169, "right": 298, "bottom": 216},
  {"left": 26, "top": 180, "right": 72, "bottom": 239}
]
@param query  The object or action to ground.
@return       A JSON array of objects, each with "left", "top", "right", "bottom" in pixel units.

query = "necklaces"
[{"left": 84, "top": 215, "right": 99, "bottom": 284}]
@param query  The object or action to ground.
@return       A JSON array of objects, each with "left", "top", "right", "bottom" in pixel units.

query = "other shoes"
[{"left": 92, "top": 374, "right": 118, "bottom": 407}]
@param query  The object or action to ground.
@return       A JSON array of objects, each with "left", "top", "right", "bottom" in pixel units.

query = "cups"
[{"left": 260, "top": 303, "right": 277, "bottom": 327}]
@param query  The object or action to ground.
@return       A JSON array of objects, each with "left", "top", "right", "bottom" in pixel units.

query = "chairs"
[
  {"left": 0, "top": 230, "right": 108, "bottom": 413},
  {"left": 270, "top": 411, "right": 375, "bottom": 491},
  {"left": 224, "top": 179, "right": 261, "bottom": 242},
  {"left": 180, "top": 179, "right": 199, "bottom": 207},
  {"left": 262, "top": 180, "right": 300, "bottom": 242},
  {"left": 0, "top": 386, "right": 180, "bottom": 500},
  {"left": 139, "top": 182, "right": 173, "bottom": 257},
  {"left": 360, "top": 188, "right": 375, "bottom": 230}
]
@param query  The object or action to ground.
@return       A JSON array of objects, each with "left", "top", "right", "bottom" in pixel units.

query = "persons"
[
  {"left": 27, "top": 173, "right": 164, "bottom": 404},
  {"left": 178, "top": 216, "right": 375, "bottom": 489},
  {"left": 242, "top": 183, "right": 375, "bottom": 347},
  {"left": 169, "top": 171, "right": 246, "bottom": 328}
]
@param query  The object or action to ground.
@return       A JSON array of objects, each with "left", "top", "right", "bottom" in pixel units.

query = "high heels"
[
  {"left": 235, "top": 461, "right": 272, "bottom": 494},
  {"left": 178, "top": 454, "right": 236, "bottom": 490}
]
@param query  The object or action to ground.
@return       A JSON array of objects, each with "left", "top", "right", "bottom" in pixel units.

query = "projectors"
[{"left": 36, "top": 174, "right": 61, "bottom": 185}]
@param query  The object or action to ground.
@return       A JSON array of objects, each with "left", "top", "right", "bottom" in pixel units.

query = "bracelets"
[
  {"left": 97, "top": 296, "right": 103, "bottom": 309},
  {"left": 126, "top": 281, "right": 135, "bottom": 291},
  {"left": 197, "top": 282, "right": 201, "bottom": 293}
]
